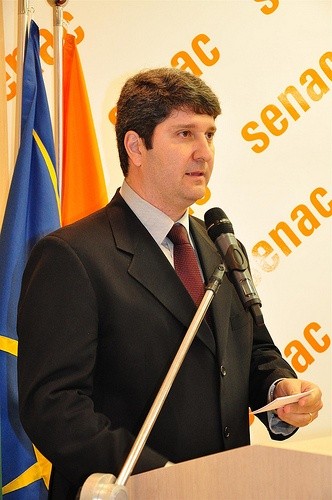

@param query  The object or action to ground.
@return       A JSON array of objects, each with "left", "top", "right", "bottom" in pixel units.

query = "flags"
[{"left": 0, "top": 19, "right": 113, "bottom": 500}]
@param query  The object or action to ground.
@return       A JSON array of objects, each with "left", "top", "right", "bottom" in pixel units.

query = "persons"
[{"left": 16, "top": 68, "right": 324, "bottom": 500}]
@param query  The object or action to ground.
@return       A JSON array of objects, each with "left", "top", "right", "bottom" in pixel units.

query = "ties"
[{"left": 166, "top": 224, "right": 215, "bottom": 336}]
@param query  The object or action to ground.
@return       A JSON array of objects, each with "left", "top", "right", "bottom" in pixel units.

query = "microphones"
[{"left": 204, "top": 207, "right": 265, "bottom": 327}]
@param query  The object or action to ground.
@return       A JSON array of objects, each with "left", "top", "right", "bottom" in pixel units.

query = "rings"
[{"left": 309, "top": 413, "right": 313, "bottom": 422}]
